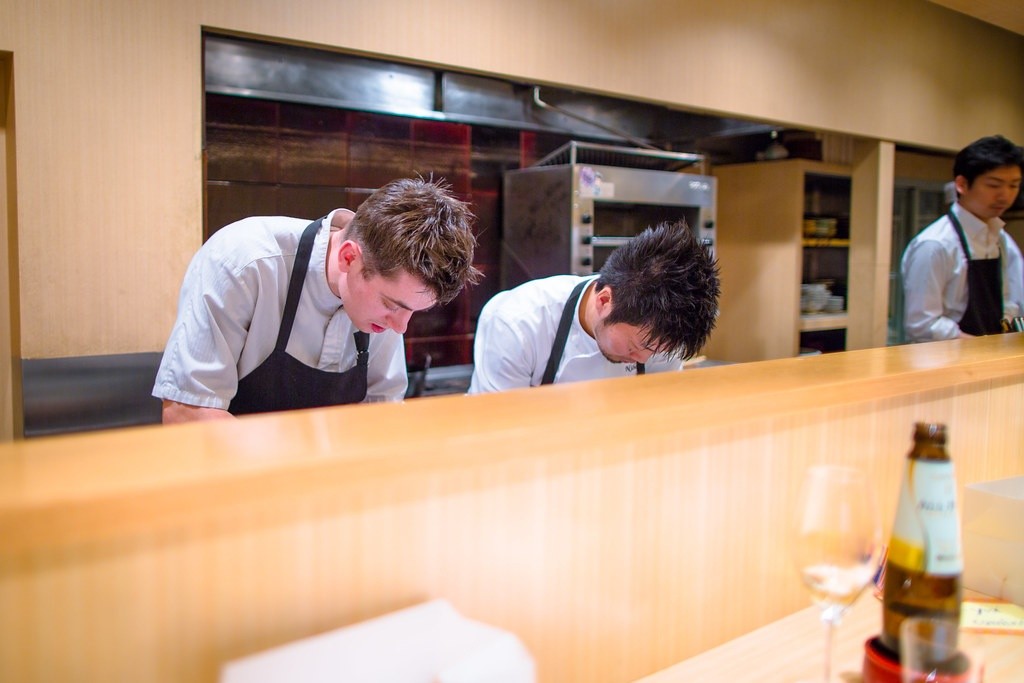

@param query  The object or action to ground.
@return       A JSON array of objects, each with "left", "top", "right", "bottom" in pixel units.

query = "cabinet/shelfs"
[{"left": 708, "top": 158, "right": 853, "bottom": 362}]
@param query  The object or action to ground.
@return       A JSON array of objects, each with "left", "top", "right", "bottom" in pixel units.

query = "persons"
[
  {"left": 902, "top": 135, "right": 1024, "bottom": 341},
  {"left": 469, "top": 222, "right": 721, "bottom": 393},
  {"left": 151, "top": 178, "right": 485, "bottom": 424}
]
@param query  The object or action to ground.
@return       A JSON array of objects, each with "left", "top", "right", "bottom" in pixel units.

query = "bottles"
[{"left": 878, "top": 423, "right": 964, "bottom": 672}]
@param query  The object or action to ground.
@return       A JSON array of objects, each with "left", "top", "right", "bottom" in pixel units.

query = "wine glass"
[{"left": 792, "top": 459, "right": 881, "bottom": 683}]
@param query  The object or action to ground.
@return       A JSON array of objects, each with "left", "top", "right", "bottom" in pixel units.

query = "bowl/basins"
[{"left": 802, "top": 277, "right": 843, "bottom": 316}]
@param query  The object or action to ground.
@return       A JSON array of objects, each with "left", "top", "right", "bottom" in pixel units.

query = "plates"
[{"left": 804, "top": 213, "right": 836, "bottom": 239}]
